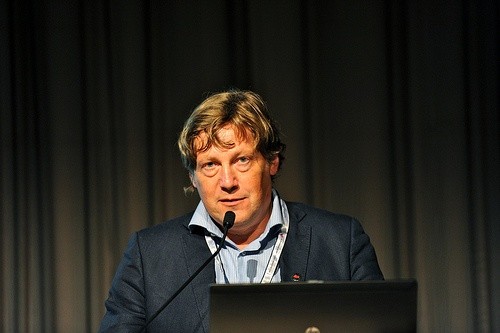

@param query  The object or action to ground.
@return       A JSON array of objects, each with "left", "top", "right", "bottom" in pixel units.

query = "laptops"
[{"left": 208, "top": 279, "right": 418, "bottom": 333}]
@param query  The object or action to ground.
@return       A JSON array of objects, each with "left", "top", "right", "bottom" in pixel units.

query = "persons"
[{"left": 100, "top": 91, "right": 383, "bottom": 333}]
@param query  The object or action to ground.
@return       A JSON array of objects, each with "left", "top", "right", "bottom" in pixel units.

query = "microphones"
[{"left": 135, "top": 210, "right": 235, "bottom": 333}]
